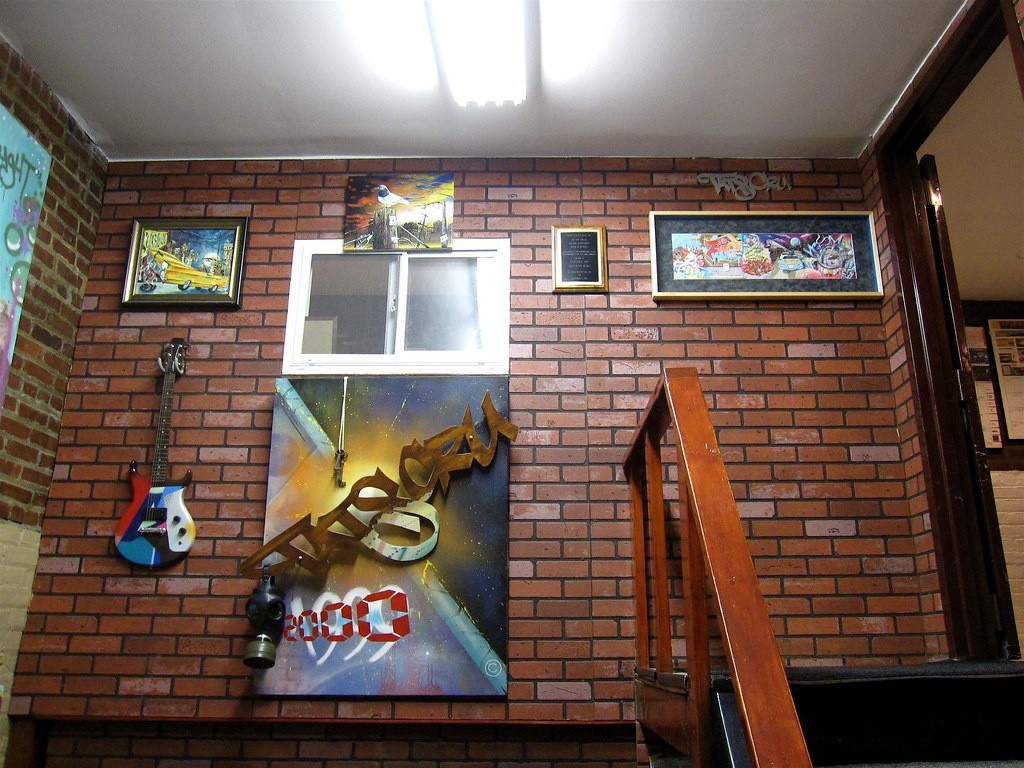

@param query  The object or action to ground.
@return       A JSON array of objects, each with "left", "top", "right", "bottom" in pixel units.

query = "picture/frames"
[
  {"left": 648, "top": 210, "right": 885, "bottom": 299},
  {"left": 120, "top": 215, "right": 249, "bottom": 309}
]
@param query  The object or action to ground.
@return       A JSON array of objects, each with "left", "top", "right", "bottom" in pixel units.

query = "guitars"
[{"left": 113, "top": 338, "right": 197, "bottom": 570}]
